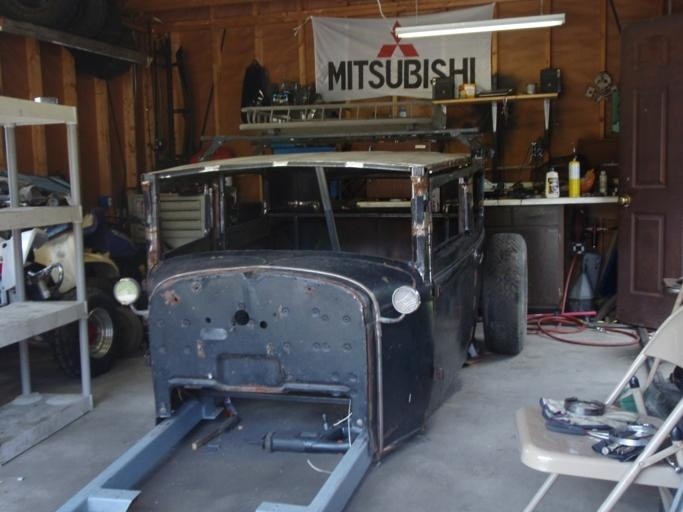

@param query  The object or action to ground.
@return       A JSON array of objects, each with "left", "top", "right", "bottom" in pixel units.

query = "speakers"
[
  {"left": 540, "top": 68, "right": 562, "bottom": 93},
  {"left": 432, "top": 76, "right": 454, "bottom": 100},
  {"left": 74, "top": 24, "right": 133, "bottom": 82}
]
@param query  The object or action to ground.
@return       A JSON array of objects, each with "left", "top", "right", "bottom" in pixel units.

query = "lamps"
[{"left": 395, "top": 1, "right": 566, "bottom": 39}]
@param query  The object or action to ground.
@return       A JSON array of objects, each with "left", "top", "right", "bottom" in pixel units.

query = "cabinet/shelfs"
[
  {"left": 0, "top": 95, "right": 94, "bottom": 466},
  {"left": 128, "top": 193, "right": 210, "bottom": 253}
]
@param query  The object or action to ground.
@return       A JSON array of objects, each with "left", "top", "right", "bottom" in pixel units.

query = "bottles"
[
  {"left": 568, "top": 157, "right": 581, "bottom": 196},
  {"left": 397, "top": 106, "right": 409, "bottom": 144},
  {"left": 599, "top": 170, "right": 607, "bottom": 193},
  {"left": 545, "top": 168, "right": 561, "bottom": 198}
]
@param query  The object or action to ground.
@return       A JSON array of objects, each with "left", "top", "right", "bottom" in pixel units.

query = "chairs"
[{"left": 515, "top": 283, "right": 683, "bottom": 512}]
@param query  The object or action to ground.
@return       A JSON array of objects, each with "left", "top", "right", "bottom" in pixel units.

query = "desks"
[{"left": 354, "top": 194, "right": 620, "bottom": 313}]
